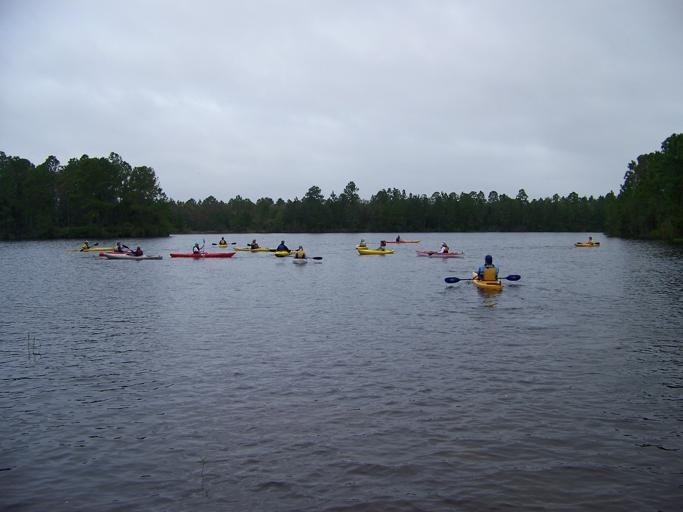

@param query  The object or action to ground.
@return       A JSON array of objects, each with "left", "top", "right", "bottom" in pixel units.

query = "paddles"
[
  {"left": 577, "top": 242, "right": 600, "bottom": 244},
  {"left": 212, "top": 242, "right": 237, "bottom": 246},
  {"left": 247, "top": 244, "right": 261, "bottom": 248},
  {"left": 275, "top": 254, "right": 322, "bottom": 260},
  {"left": 445, "top": 275, "right": 521, "bottom": 283},
  {"left": 80, "top": 243, "right": 98, "bottom": 252},
  {"left": 122, "top": 245, "right": 134, "bottom": 253}
]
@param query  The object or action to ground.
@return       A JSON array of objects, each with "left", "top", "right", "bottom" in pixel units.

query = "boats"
[
  {"left": 218, "top": 244, "right": 228, "bottom": 248},
  {"left": 169, "top": 251, "right": 236, "bottom": 258},
  {"left": 575, "top": 242, "right": 601, "bottom": 247},
  {"left": 415, "top": 250, "right": 465, "bottom": 258},
  {"left": 355, "top": 244, "right": 395, "bottom": 255},
  {"left": 64, "top": 247, "right": 162, "bottom": 260},
  {"left": 233, "top": 247, "right": 307, "bottom": 264},
  {"left": 471, "top": 271, "right": 503, "bottom": 291},
  {"left": 386, "top": 240, "right": 420, "bottom": 243}
]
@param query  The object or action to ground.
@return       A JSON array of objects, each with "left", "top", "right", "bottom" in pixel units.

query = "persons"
[
  {"left": 219, "top": 237, "right": 228, "bottom": 245},
  {"left": 82, "top": 240, "right": 91, "bottom": 249},
  {"left": 377, "top": 241, "right": 386, "bottom": 251},
  {"left": 130, "top": 246, "right": 143, "bottom": 256},
  {"left": 114, "top": 242, "right": 124, "bottom": 252},
  {"left": 250, "top": 239, "right": 261, "bottom": 249},
  {"left": 437, "top": 242, "right": 449, "bottom": 253},
  {"left": 360, "top": 239, "right": 366, "bottom": 247},
  {"left": 192, "top": 241, "right": 207, "bottom": 254},
  {"left": 586, "top": 236, "right": 594, "bottom": 245},
  {"left": 294, "top": 245, "right": 305, "bottom": 259},
  {"left": 477, "top": 254, "right": 498, "bottom": 281},
  {"left": 276, "top": 240, "right": 290, "bottom": 253}
]
